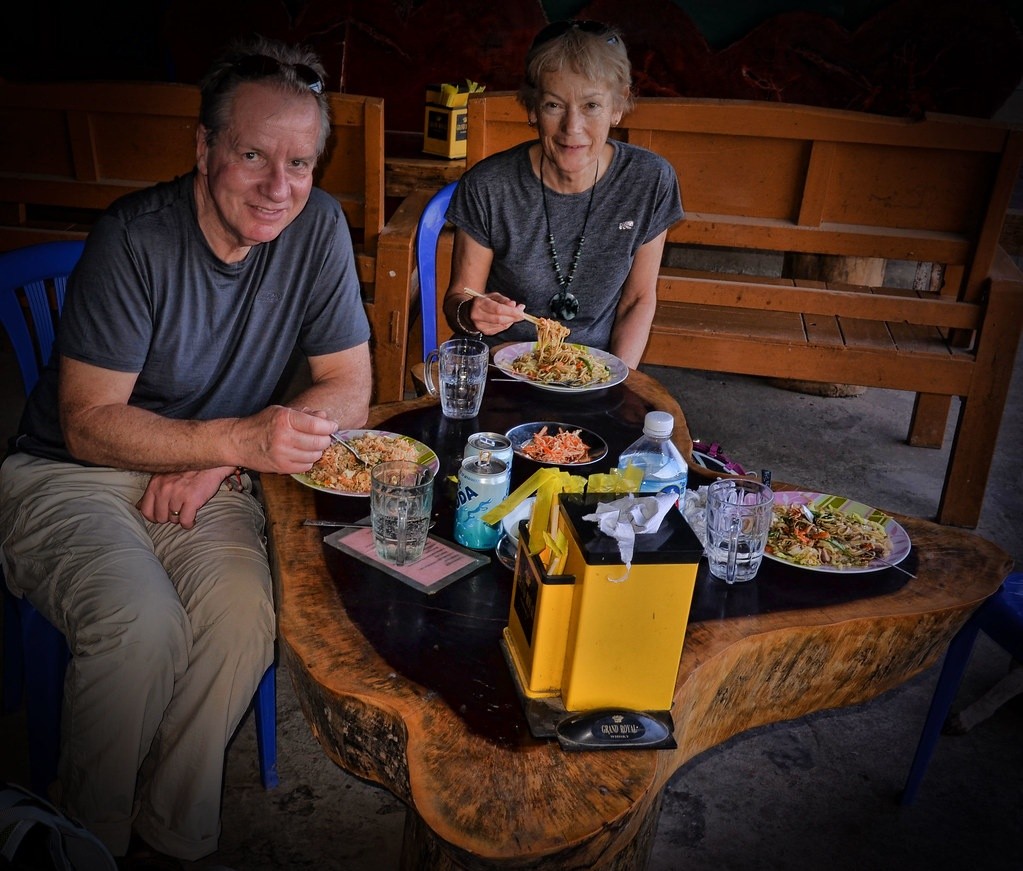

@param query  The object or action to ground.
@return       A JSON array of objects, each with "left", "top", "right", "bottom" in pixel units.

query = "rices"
[{"left": 304, "top": 432, "right": 420, "bottom": 492}]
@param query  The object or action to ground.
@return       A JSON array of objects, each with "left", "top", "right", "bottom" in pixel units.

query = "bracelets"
[{"left": 456, "top": 298, "right": 483, "bottom": 341}]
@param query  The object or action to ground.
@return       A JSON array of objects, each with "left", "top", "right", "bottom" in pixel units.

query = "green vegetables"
[
  {"left": 766, "top": 509, "right": 855, "bottom": 557},
  {"left": 535, "top": 343, "right": 609, "bottom": 375}
]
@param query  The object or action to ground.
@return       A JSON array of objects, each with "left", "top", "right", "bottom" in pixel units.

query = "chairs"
[
  {"left": 895, "top": 571, "right": 1023, "bottom": 814},
  {"left": 0, "top": 239, "right": 281, "bottom": 793},
  {"left": 415, "top": 181, "right": 460, "bottom": 362}
]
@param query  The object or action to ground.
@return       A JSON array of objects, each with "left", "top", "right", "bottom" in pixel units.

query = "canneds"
[{"left": 452, "top": 432, "right": 514, "bottom": 550}]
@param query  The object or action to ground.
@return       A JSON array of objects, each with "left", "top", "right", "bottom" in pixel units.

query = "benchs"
[
  {"left": 432, "top": 93, "right": 1023, "bottom": 531},
  {"left": 0, "top": 84, "right": 443, "bottom": 406}
]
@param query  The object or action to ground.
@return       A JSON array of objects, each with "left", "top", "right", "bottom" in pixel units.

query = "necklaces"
[{"left": 540, "top": 154, "right": 598, "bottom": 320}]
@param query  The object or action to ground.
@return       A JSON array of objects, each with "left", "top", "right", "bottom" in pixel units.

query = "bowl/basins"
[{"left": 502, "top": 497, "right": 539, "bottom": 548}]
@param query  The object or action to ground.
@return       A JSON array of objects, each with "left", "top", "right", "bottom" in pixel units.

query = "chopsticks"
[{"left": 464, "top": 288, "right": 569, "bottom": 336}]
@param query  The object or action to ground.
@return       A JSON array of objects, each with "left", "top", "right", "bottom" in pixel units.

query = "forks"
[{"left": 490, "top": 377, "right": 600, "bottom": 388}]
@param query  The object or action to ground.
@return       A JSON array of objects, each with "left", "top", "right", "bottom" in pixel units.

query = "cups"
[
  {"left": 370, "top": 460, "right": 434, "bottom": 567},
  {"left": 424, "top": 339, "right": 489, "bottom": 419},
  {"left": 707, "top": 478, "right": 776, "bottom": 584}
]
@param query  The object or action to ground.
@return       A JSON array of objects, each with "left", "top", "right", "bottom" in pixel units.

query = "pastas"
[
  {"left": 765, "top": 503, "right": 890, "bottom": 567},
  {"left": 513, "top": 316, "right": 609, "bottom": 386}
]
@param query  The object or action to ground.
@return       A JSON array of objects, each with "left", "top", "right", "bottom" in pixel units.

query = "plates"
[
  {"left": 493, "top": 341, "right": 629, "bottom": 392},
  {"left": 290, "top": 429, "right": 440, "bottom": 497},
  {"left": 742, "top": 491, "right": 911, "bottom": 573},
  {"left": 506, "top": 421, "right": 609, "bottom": 466}
]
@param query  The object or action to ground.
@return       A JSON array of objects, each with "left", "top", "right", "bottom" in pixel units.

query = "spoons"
[
  {"left": 802, "top": 503, "right": 917, "bottom": 579},
  {"left": 329, "top": 433, "right": 376, "bottom": 465}
]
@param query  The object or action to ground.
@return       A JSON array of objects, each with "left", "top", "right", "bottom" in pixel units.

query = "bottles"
[{"left": 618, "top": 411, "right": 688, "bottom": 517}]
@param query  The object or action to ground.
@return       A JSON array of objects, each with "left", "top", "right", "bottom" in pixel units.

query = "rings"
[{"left": 169, "top": 511, "right": 179, "bottom": 515}]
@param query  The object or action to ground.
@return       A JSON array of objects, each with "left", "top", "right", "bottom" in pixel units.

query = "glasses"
[
  {"left": 526, "top": 19, "right": 618, "bottom": 67},
  {"left": 225, "top": 53, "right": 327, "bottom": 95}
]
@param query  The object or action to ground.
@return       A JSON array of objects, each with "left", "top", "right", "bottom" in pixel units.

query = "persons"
[
  {"left": 0, "top": 48, "right": 371, "bottom": 870},
  {"left": 442, "top": 19, "right": 684, "bottom": 370}
]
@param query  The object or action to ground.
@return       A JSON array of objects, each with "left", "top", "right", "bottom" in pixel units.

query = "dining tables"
[{"left": 248, "top": 367, "right": 1015, "bottom": 871}]
